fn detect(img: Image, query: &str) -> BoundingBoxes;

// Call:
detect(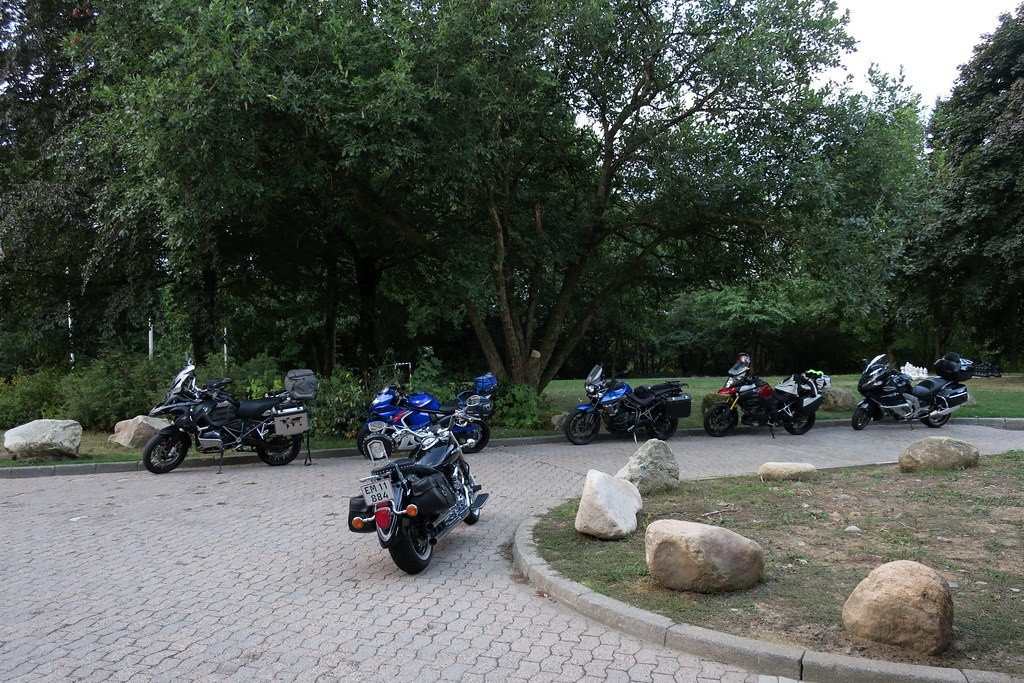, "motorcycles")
[850,348,976,431]
[141,357,319,474]
[563,362,691,445]
[347,392,490,575]
[355,371,515,459]
[703,352,831,438]
